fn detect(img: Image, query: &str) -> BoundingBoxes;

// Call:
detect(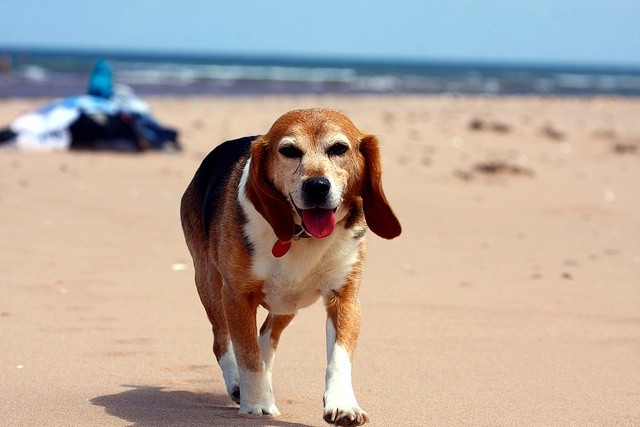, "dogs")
[179,107,402,427]
